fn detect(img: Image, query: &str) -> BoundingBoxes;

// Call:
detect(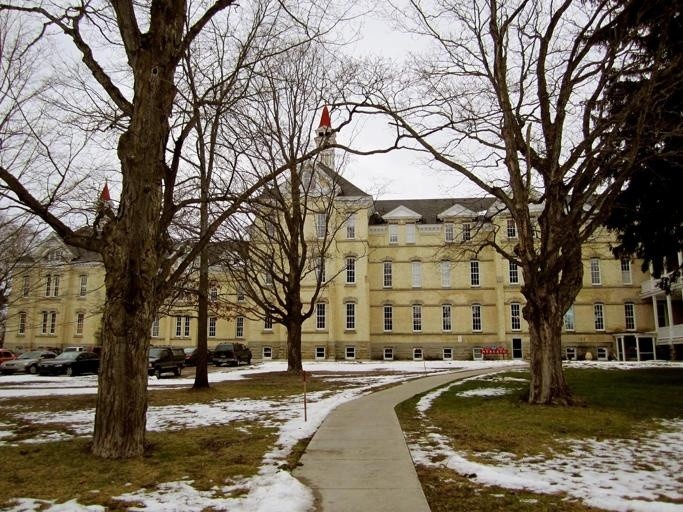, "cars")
[148,342,252,379]
[0,347,100,377]
